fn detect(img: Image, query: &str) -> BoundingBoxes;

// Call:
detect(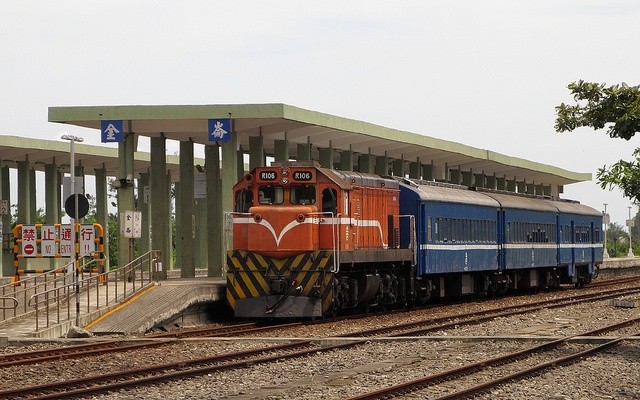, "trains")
[226,161,606,321]
